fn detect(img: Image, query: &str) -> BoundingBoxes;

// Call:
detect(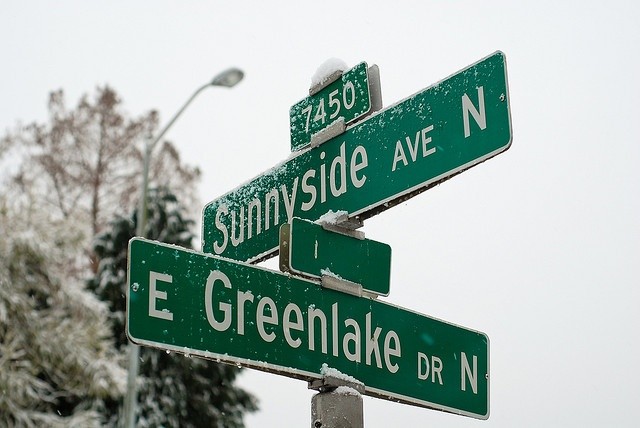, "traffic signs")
[202,51,513,266]
[126,216,492,421]
